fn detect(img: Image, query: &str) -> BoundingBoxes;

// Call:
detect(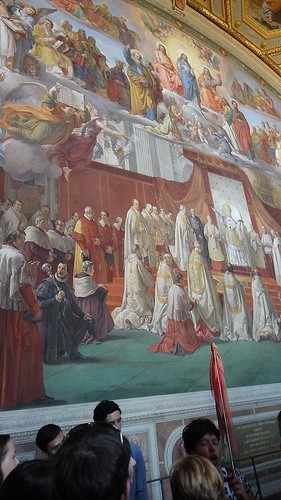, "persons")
[93,400,148,500]
[169,455,226,500]
[35,424,65,457]
[0,434,19,484]
[0,459,54,500]
[54,422,137,500]
[182,418,256,500]
